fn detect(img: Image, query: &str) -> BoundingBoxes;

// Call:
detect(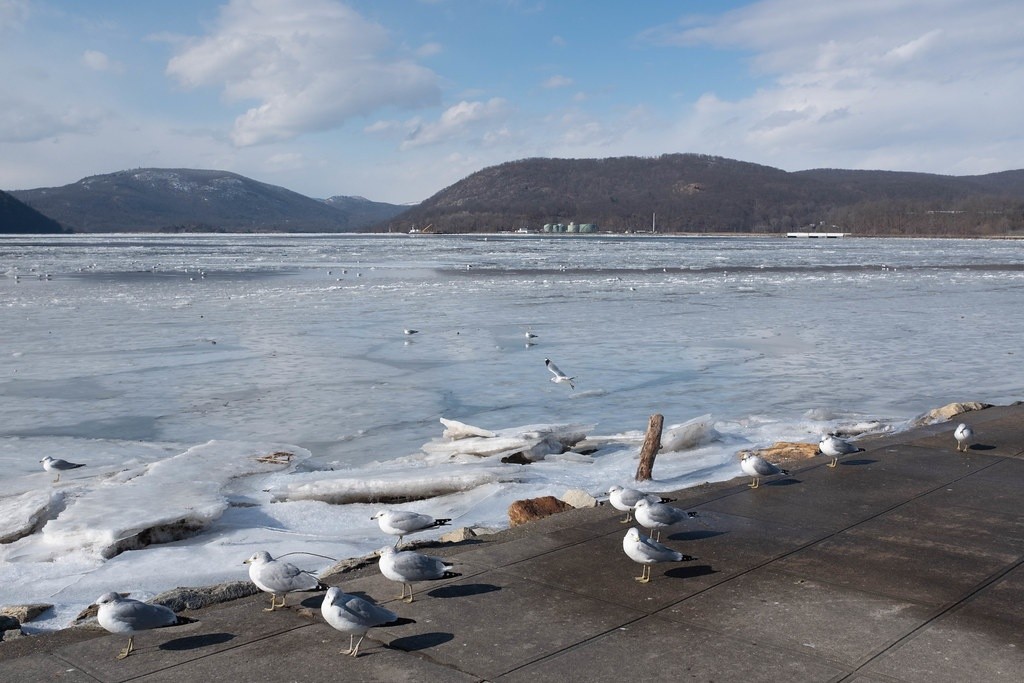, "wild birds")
[954,423,975,452]
[603,484,698,543]
[374,545,464,604]
[623,528,692,584]
[525,330,539,342]
[38,455,87,484]
[321,587,417,658]
[87,592,199,660]
[370,507,453,549]
[739,450,795,489]
[543,357,577,381]
[402,327,420,336]
[819,434,867,468]
[242,551,328,612]
